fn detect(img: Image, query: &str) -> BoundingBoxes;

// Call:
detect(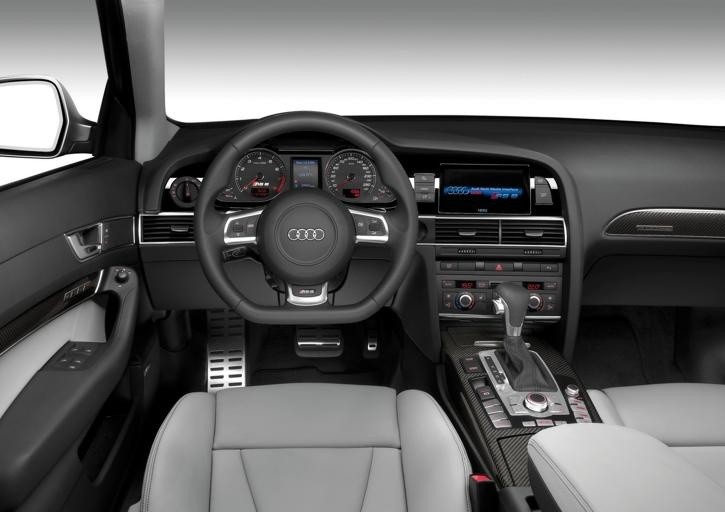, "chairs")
[128,380,474,512]
[583,376,725,489]
